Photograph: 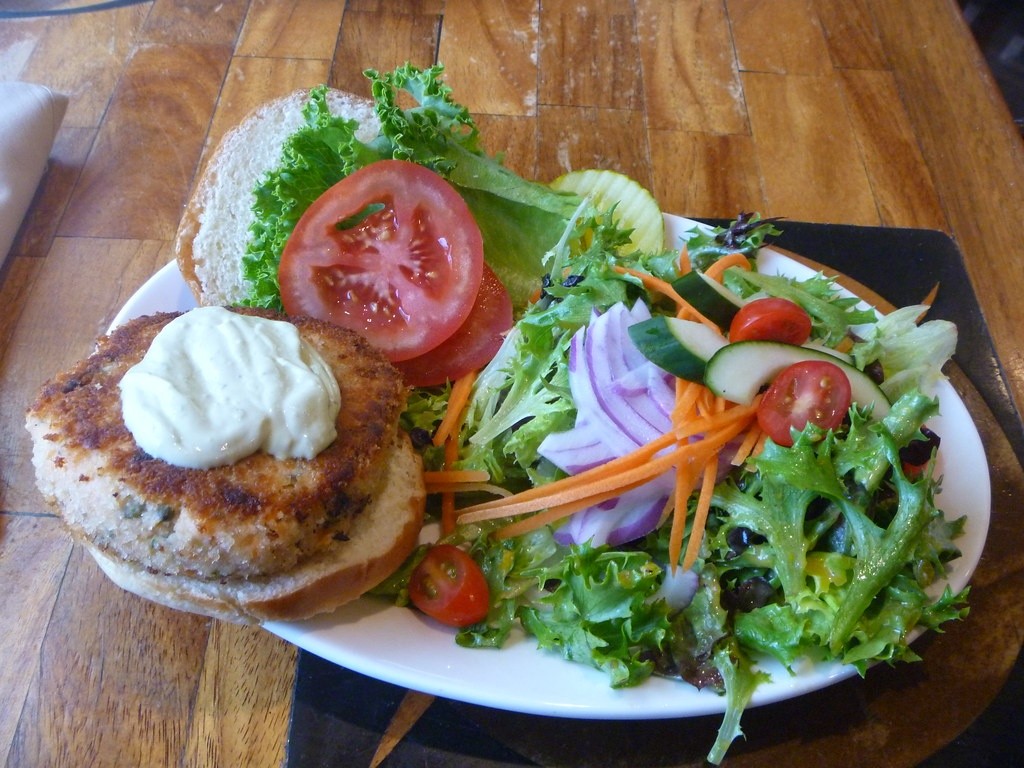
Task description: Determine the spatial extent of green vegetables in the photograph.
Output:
[244,57,973,767]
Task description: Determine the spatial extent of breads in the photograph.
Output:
[175,84,385,308]
[26,301,427,625]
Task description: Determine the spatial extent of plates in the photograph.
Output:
[87,196,996,717]
[274,223,1023,768]
